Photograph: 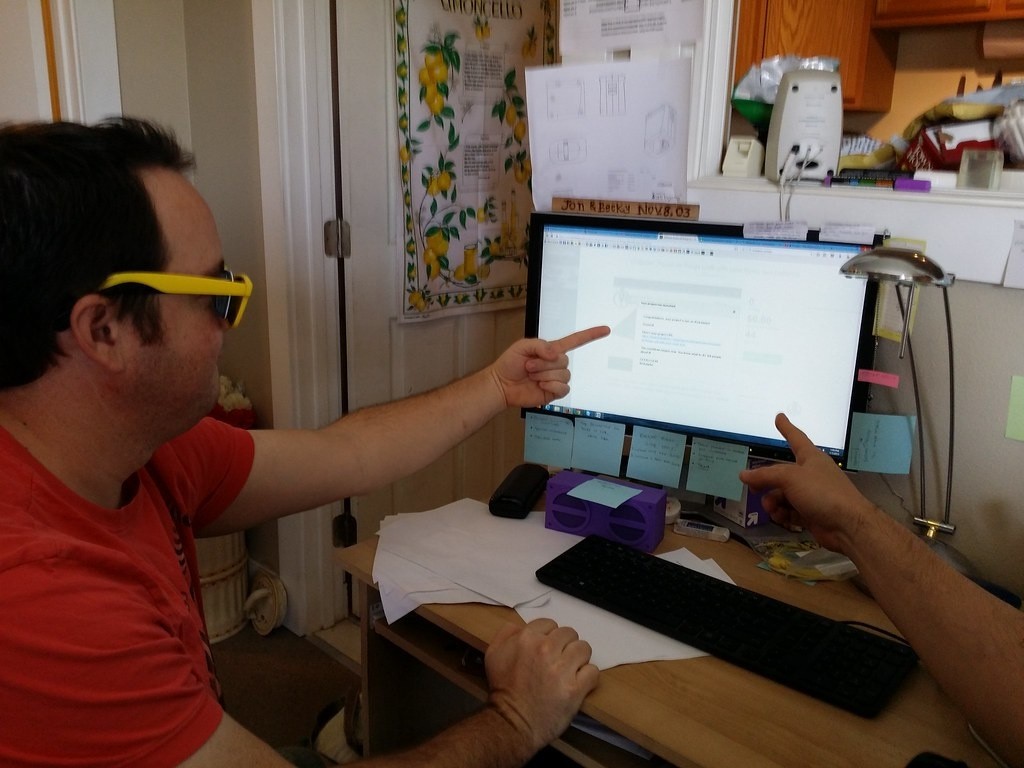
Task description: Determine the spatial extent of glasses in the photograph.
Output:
[99,263,253,329]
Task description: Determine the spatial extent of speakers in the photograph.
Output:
[546,472,666,552]
[765,69,845,181]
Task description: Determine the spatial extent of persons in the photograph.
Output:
[739,412,1023,768]
[1,112,612,768]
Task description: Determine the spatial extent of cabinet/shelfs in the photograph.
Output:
[733,1,1024,112]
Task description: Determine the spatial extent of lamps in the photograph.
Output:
[841,247,959,543]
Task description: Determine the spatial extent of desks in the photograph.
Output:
[333,499,1007,768]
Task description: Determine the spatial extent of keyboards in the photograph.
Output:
[536,534,920,720]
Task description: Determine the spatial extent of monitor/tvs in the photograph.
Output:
[522,212,891,474]
